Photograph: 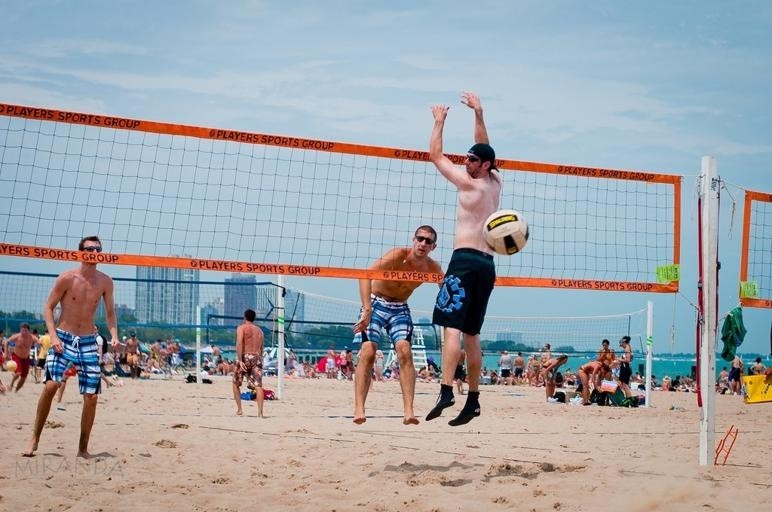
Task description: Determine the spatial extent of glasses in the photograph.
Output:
[467,154,479,162]
[84,246,102,252]
[415,236,435,244]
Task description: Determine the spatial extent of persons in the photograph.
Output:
[0,325,765,407]
[233,309,264,418]
[426,91,503,426]
[21,236,119,459]
[352,225,446,425]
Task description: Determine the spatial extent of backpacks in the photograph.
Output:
[95,335,107,354]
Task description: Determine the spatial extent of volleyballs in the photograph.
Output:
[483,209,529,255]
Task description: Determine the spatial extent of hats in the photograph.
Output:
[468,144,499,172]
